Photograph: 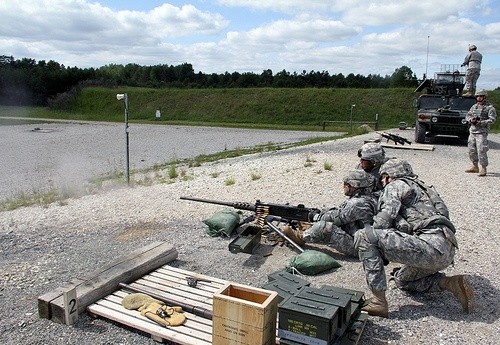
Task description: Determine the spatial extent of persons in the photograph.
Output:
[465,88,496,177]
[285,142,396,260]
[444,45,483,97]
[353,158,475,318]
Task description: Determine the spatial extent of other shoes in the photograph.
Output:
[461,91,473,96]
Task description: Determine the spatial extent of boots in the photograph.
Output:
[360,289,389,318]
[297,221,311,230]
[465,162,479,173]
[284,227,305,245]
[439,275,474,314]
[478,165,486,176]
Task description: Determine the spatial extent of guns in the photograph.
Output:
[179,195,320,226]
[376,130,412,146]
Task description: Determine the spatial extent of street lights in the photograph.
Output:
[425,35,431,79]
[116,93,132,188]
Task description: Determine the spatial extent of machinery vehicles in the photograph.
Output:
[410,63,480,144]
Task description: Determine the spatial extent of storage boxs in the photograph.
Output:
[212,270,366,345]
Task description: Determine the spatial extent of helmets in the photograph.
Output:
[475,88,488,97]
[469,45,476,51]
[380,158,413,179]
[342,171,369,196]
[358,143,384,165]
